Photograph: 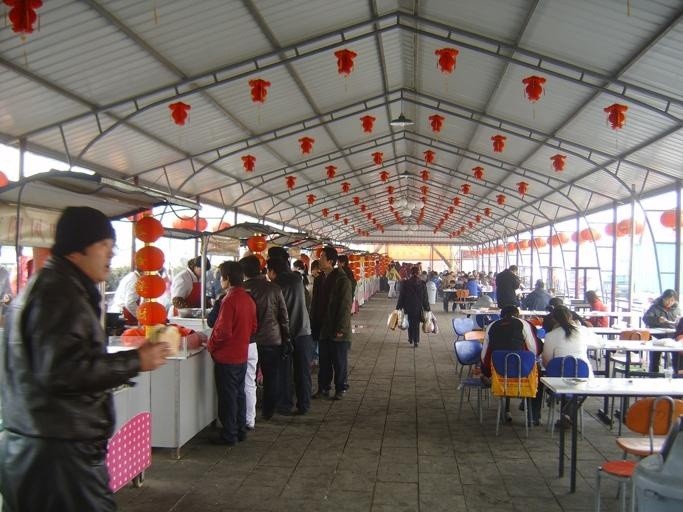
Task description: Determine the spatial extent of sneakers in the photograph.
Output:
[555,418,572,429]
[506,410,512,421]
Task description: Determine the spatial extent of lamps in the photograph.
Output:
[387,89,414,130]
[397,155,414,177]
[391,188,424,235]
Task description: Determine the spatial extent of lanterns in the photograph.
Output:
[3,0,43,33]
[248,79,271,105]
[360,115,375,133]
[0,170,9,189]
[522,76,545,103]
[428,115,444,133]
[335,48,357,79]
[435,48,459,74]
[604,104,627,130]
[169,102,191,126]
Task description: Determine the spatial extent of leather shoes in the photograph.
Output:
[207,429,245,446]
[408,339,418,347]
[334,388,346,400]
[311,389,330,400]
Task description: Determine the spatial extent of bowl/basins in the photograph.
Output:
[175,307,202,318]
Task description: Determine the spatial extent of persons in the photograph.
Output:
[0,207,175,512]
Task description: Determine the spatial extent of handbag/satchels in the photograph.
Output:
[423,310,434,333]
[386,308,409,331]
[420,305,426,323]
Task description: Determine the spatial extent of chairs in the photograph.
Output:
[490,348,538,444]
[540,317,683,510]
[455,342,494,423]
[426,275,640,342]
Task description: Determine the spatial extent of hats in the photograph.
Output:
[50,206,116,258]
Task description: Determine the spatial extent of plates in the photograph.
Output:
[563,378,587,386]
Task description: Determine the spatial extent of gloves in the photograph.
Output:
[279,334,294,360]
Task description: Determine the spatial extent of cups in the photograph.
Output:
[663,369,673,383]
